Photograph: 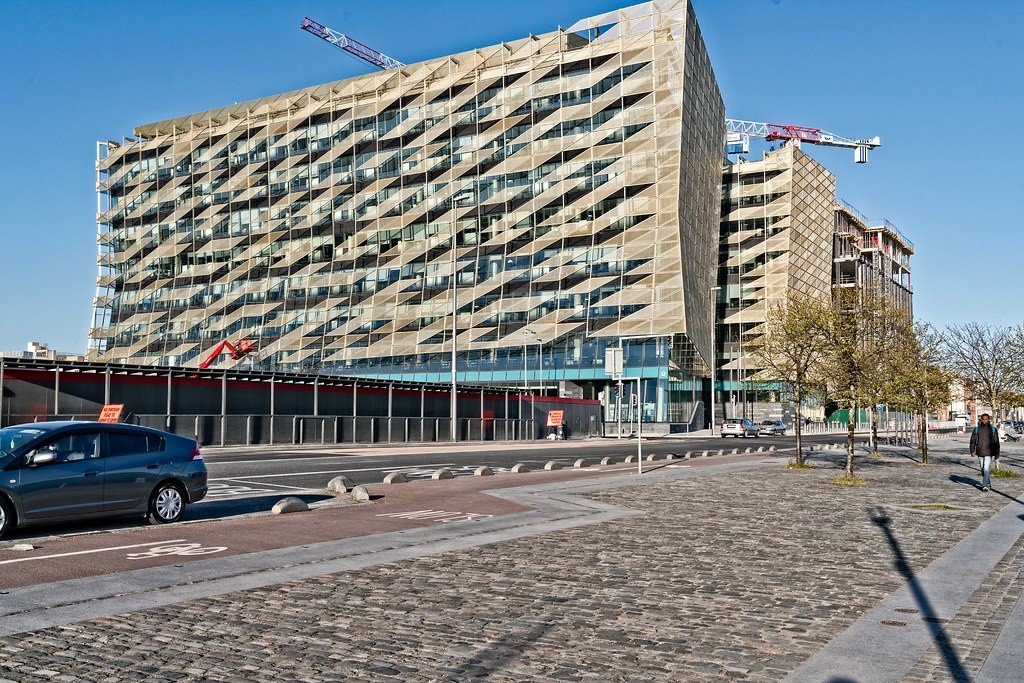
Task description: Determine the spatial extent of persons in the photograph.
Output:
[806,417,813,425]
[1018,419,1024,427]
[970,414,1000,492]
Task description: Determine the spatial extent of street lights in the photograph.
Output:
[710,286,722,435]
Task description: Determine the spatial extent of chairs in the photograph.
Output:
[63,435,91,462]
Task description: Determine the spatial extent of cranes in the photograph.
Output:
[724,118,881,163]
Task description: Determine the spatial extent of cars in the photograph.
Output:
[955,415,970,427]
[759,420,787,436]
[0,421,210,541]
[720,418,760,439]
[1001,420,1024,429]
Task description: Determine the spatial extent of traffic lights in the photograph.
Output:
[633,395,636,405]
[615,384,620,397]
[622,384,626,398]
[668,335,673,349]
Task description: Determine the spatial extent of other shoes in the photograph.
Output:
[983,484,991,492]
